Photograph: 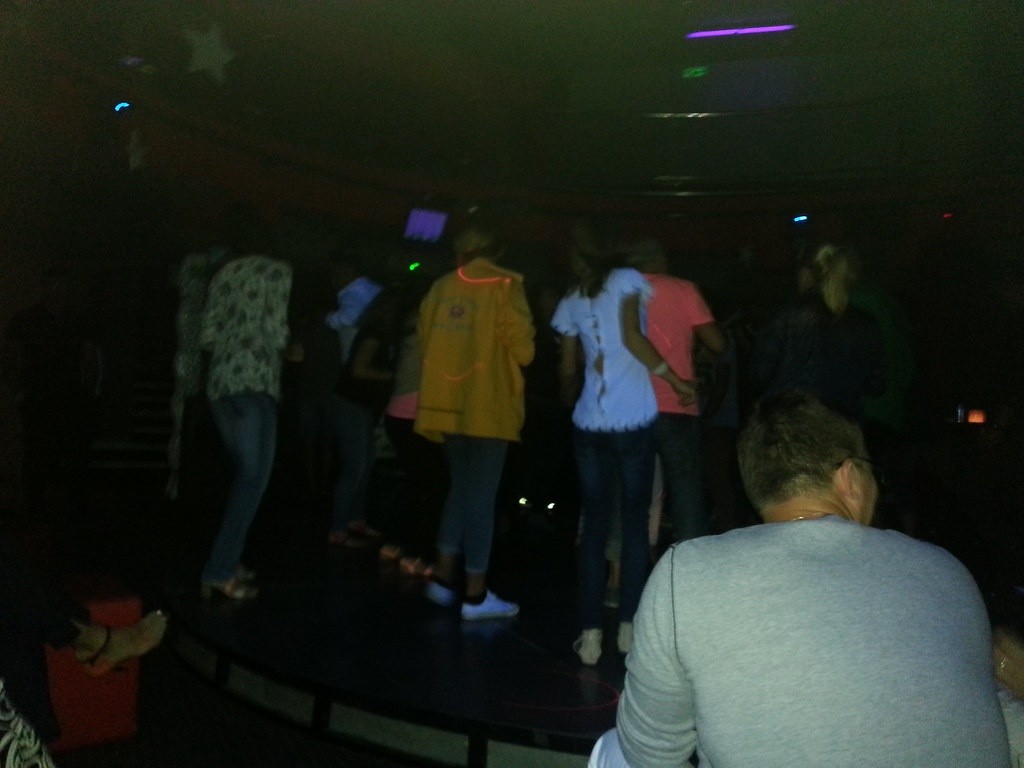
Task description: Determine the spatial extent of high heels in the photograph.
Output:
[201,579,257,600]
[234,566,253,580]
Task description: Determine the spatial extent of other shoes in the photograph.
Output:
[617,621,633,653]
[571,629,603,664]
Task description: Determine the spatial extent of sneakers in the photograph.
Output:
[461,590,520,621]
[421,576,453,607]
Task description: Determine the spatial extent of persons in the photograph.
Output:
[0,215,1024,768]
[584,380,1010,767]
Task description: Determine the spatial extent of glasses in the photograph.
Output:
[840,457,881,482]
